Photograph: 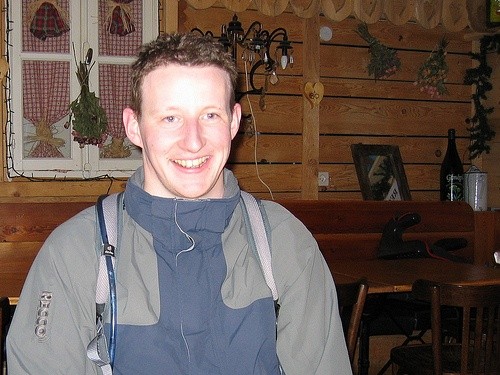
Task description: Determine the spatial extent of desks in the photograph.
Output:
[327,260,500,294]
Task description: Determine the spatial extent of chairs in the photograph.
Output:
[336,284,369,356]
[391,279,500,375]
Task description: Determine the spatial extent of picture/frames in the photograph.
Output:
[350,143,412,201]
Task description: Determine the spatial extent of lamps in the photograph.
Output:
[189,14,294,110]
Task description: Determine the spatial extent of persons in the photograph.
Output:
[5,32,353,375]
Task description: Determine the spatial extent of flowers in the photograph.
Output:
[355,23,401,82]
[413,36,451,98]
[63,43,111,148]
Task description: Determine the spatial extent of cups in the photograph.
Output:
[463,173,488,211]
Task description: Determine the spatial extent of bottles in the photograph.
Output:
[439,128,464,202]
